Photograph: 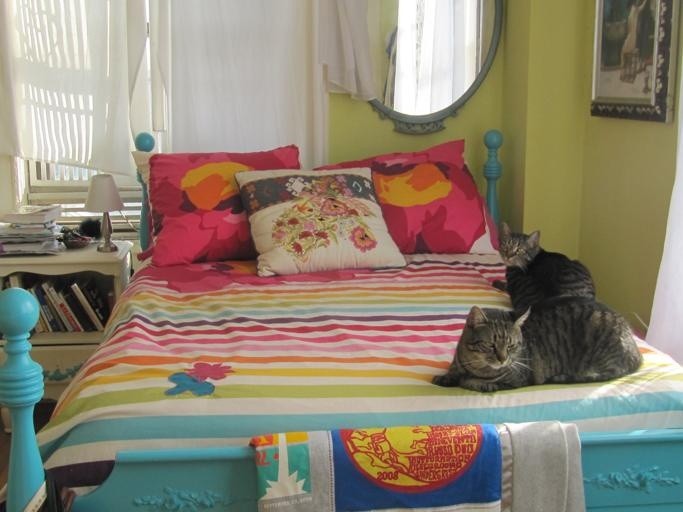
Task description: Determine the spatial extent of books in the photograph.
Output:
[0,273,114,333]
[0,204,59,255]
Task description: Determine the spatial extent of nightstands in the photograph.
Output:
[0,239,135,433]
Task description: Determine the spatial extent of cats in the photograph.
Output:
[491,222,596,311]
[431,295,643,393]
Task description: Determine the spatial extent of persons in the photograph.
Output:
[619,0,648,70]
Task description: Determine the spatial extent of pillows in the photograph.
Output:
[234,167,406,278]
[131,144,301,267]
[313,138,501,256]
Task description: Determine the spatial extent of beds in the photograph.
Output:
[0,128,683,512]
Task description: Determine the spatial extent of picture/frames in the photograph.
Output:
[589,0,680,126]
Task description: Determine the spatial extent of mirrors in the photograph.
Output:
[358,0,504,135]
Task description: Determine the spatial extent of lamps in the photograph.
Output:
[83,173,126,253]
